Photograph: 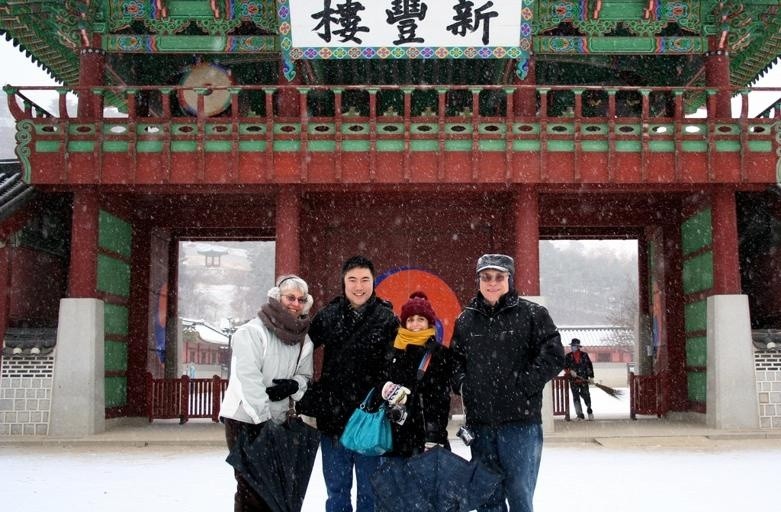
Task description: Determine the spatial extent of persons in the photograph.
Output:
[446,252,567,512]
[564,338,596,421]
[359,291,451,461]
[218,275,314,512]
[307,254,407,511]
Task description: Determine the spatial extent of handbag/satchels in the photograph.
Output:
[339,387,394,458]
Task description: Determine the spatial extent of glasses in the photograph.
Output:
[478,273,509,282]
[280,293,308,304]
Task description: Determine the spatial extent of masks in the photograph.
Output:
[572,345,577,352]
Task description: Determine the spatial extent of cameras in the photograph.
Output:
[456,426,476,447]
[390,404,409,426]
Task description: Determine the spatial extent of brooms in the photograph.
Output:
[575,374,625,401]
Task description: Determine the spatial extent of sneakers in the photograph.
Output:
[575,414,594,422]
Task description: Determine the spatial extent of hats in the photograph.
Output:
[401,291,436,329]
[569,338,583,348]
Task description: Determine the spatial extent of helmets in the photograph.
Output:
[475,253,515,274]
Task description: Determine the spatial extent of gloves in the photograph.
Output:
[590,378,594,384]
[571,370,576,377]
[265,379,299,402]
[382,381,412,426]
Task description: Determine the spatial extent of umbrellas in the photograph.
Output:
[222,392,325,512]
[366,393,507,512]
